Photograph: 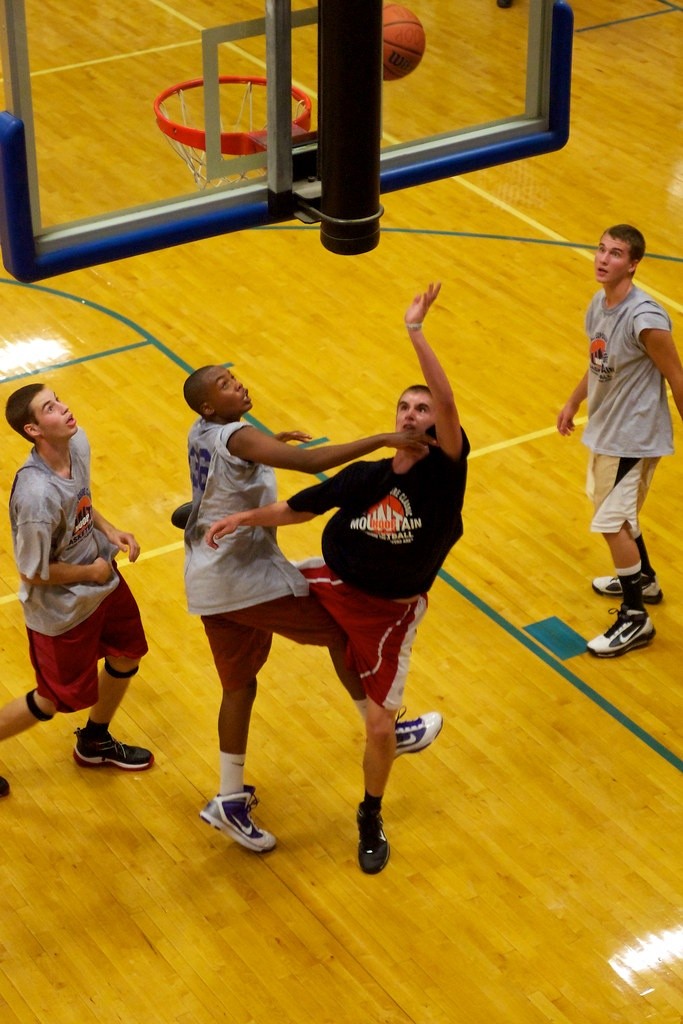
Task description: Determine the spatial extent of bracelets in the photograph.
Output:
[409,323,421,329]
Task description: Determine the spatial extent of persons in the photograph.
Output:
[0,384,155,798]
[556,224,683,657]
[182,366,443,854]
[170,283,472,875]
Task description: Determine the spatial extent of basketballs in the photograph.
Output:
[382,3,426,81]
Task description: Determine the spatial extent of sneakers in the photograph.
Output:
[592,571,663,605]
[357,802,390,875]
[393,706,443,760]
[171,501,192,529]
[0,776,10,797]
[199,784,276,853]
[586,604,656,657]
[73,726,154,771]
